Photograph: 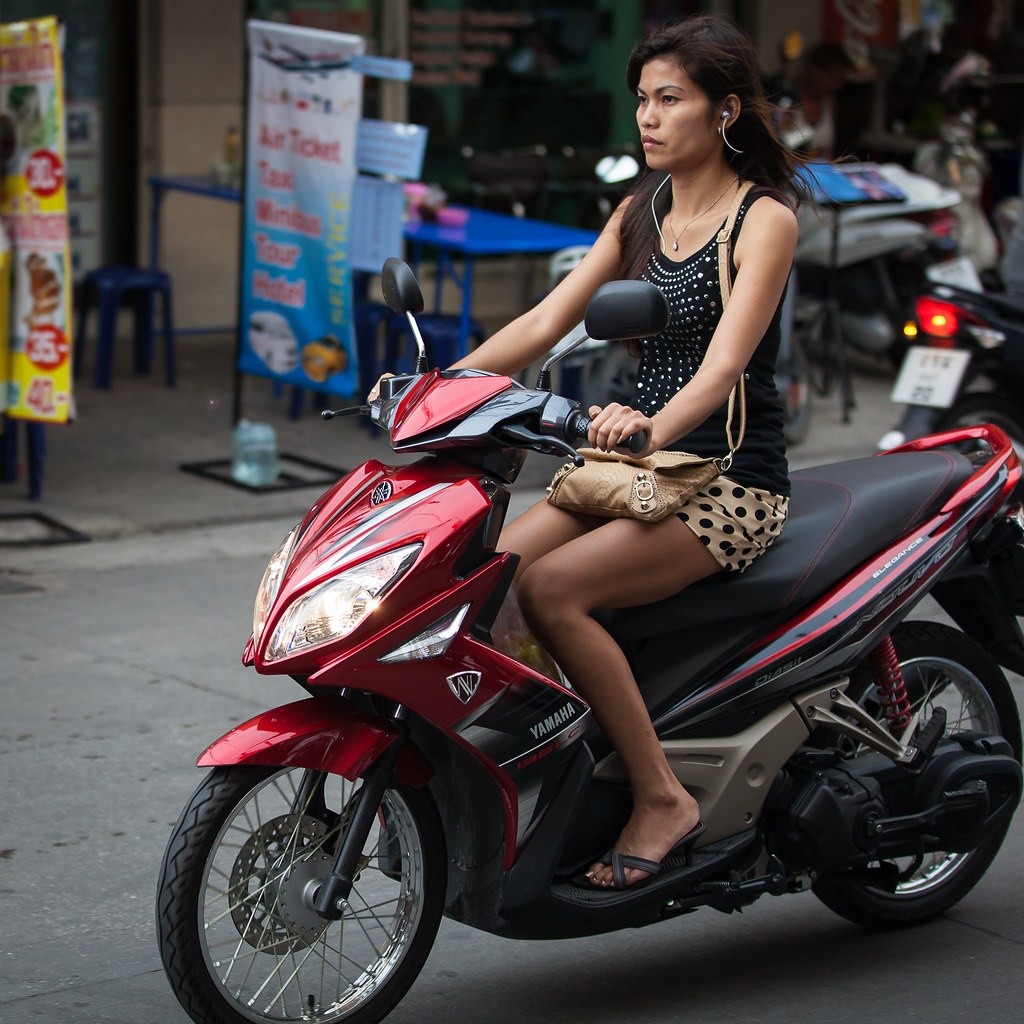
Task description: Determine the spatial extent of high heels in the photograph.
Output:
[574,807,708,890]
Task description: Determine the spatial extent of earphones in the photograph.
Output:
[721,110,730,119]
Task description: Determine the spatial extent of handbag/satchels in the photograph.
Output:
[548,440,724,524]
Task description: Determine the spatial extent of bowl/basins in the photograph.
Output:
[438,209,466,225]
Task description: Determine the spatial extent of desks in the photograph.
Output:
[142,175,599,420]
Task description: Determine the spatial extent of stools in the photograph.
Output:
[0,262,486,501]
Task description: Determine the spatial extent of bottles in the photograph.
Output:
[230,421,280,487]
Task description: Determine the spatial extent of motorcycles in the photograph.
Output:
[153,257,1024,1024]
[878,264,1024,519]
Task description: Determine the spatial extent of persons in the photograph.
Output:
[770,0,1024,353]
[461,36,614,148]
[367,17,864,889]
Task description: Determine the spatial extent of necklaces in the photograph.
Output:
[669,177,738,251]
